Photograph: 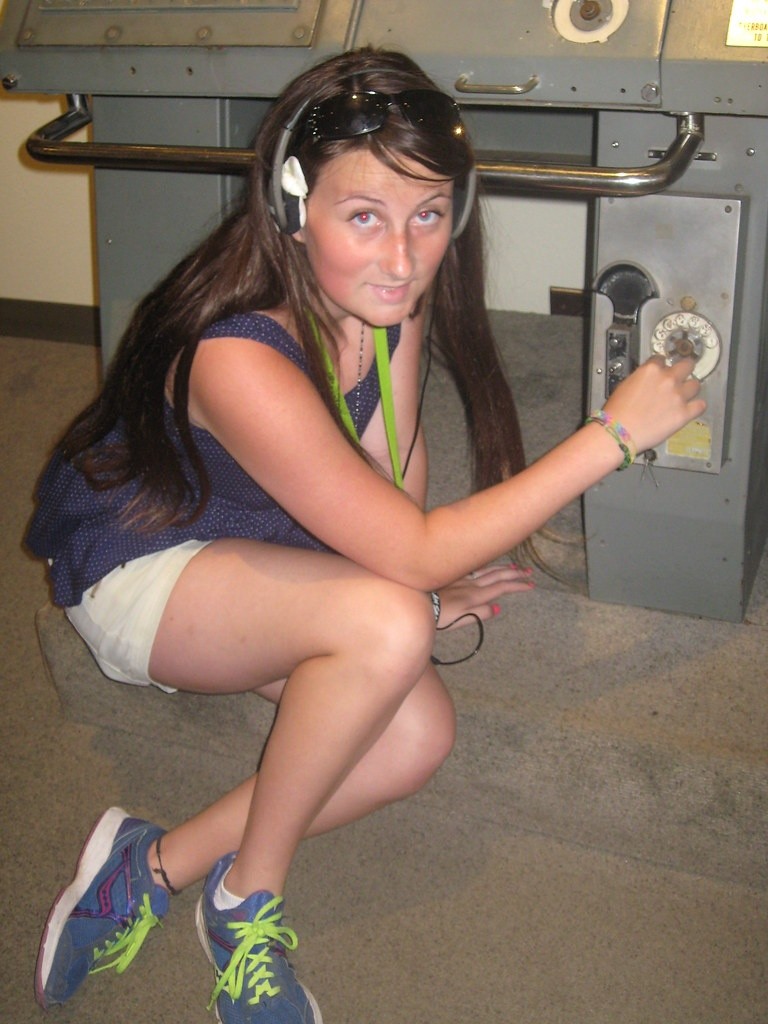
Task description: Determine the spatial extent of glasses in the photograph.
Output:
[283,89,462,159]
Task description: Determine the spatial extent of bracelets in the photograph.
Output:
[585,410,637,472]
[431,590,442,618]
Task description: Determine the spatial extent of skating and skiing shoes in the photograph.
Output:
[35,806,171,1013]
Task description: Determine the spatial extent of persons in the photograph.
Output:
[22,48,707,1024]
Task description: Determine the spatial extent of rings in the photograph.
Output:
[471,572,477,579]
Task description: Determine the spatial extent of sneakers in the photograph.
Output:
[195,850,323,1024]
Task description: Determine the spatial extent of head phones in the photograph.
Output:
[264,66,476,239]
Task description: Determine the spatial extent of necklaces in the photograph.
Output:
[351,316,365,435]
